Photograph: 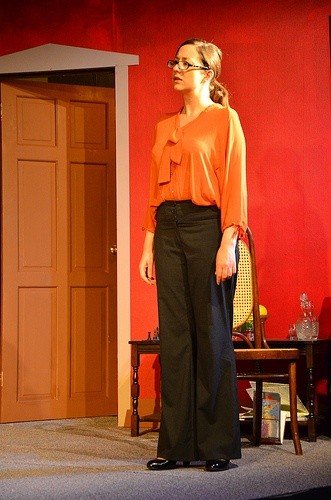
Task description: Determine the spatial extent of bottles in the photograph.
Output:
[296,291,313,342]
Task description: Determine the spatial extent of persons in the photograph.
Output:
[139,38,248,471]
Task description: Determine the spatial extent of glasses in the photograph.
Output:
[167,60,209,70]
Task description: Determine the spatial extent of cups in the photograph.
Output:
[312,321,319,341]
[288,323,299,341]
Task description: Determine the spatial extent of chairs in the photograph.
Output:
[233,227,304,456]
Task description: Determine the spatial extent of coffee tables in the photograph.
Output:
[127,340,331,447]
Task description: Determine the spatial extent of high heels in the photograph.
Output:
[147,458,191,470]
[205,459,229,471]
